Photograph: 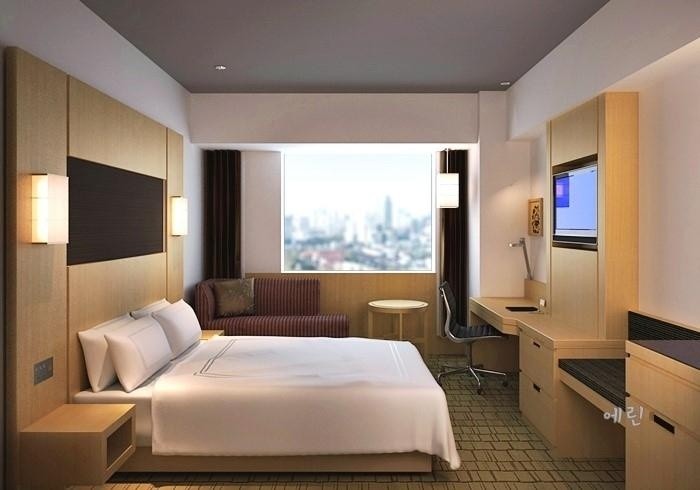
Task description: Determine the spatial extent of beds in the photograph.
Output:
[72,336,432,473]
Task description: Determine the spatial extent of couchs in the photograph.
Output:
[194,278,348,338]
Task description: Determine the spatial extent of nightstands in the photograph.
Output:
[200,328,224,340]
[18,404,137,489]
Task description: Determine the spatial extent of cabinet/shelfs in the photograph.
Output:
[517,316,627,460]
[624,337,700,489]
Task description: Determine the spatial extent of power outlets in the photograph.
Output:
[33,356,54,385]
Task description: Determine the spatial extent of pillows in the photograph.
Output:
[77,312,138,393]
[104,313,175,393]
[213,278,254,317]
[151,298,203,361]
[130,298,171,320]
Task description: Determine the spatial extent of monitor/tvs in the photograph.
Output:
[550,154,598,251]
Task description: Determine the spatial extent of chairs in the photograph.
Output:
[436,281,510,395]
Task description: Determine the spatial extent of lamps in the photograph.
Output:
[215,65,226,70]
[500,81,510,86]
[171,196,188,236]
[437,147,460,208]
[31,172,69,244]
[509,237,531,279]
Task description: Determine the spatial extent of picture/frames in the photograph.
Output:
[528,198,543,237]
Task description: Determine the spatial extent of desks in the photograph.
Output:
[368,299,428,357]
[471,296,542,335]
